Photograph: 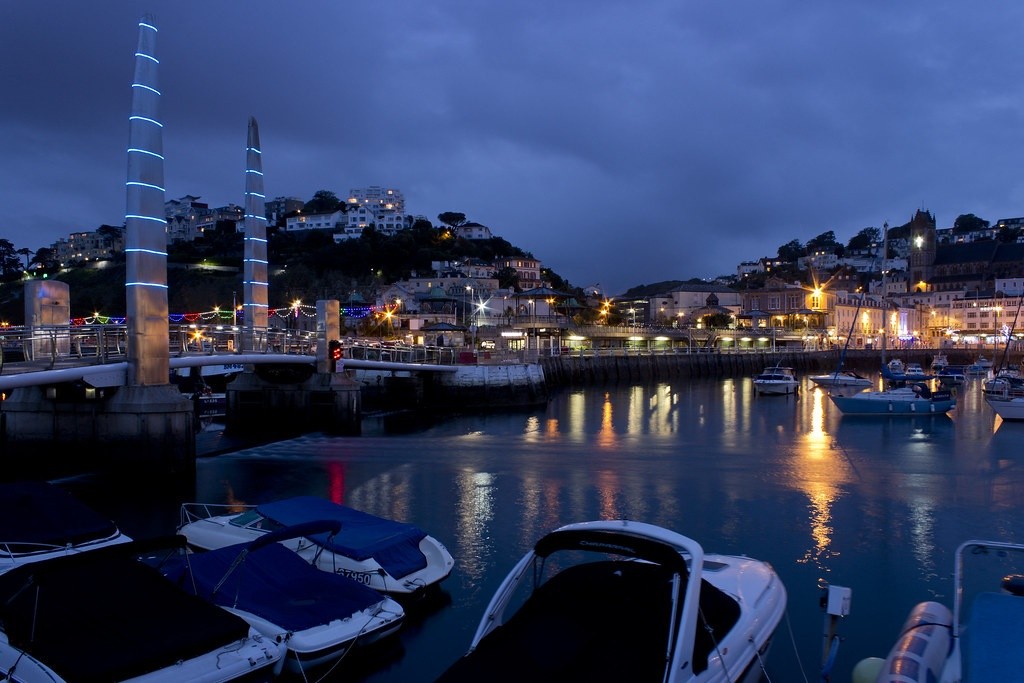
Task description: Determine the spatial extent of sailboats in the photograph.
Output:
[826,222,955,416]
[983,284,1024,424]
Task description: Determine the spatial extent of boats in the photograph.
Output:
[848,539,1023,683]
[177,498,455,603]
[0,468,291,683]
[426,514,787,683]
[752,365,799,395]
[139,537,407,675]
[810,369,872,387]
[880,354,994,387]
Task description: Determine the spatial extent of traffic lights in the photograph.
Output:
[330,340,342,361]
